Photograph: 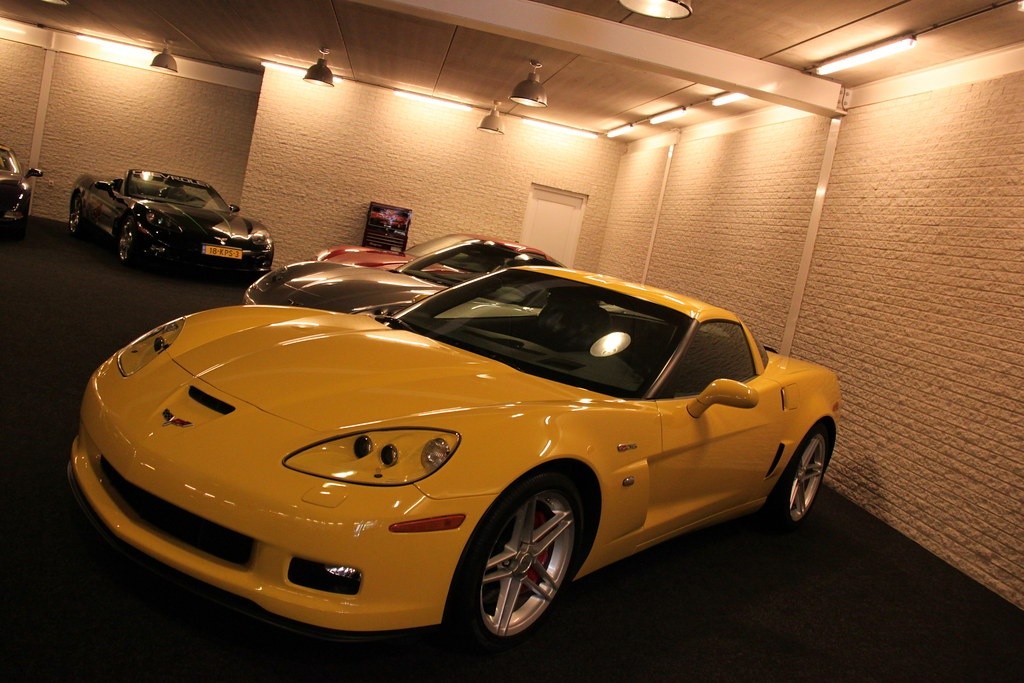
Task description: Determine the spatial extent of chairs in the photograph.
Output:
[109,179,143,196]
[159,187,192,202]
[462,248,496,269]
[539,296,612,358]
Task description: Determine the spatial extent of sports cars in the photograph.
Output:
[69,169,275,272]
[244,232,626,320]
[68,264,840,654]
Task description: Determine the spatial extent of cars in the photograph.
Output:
[0,145,43,239]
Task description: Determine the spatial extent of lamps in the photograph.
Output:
[508,59,548,108]
[478,98,504,136]
[565,306,633,357]
[616,0,693,20]
[149,40,178,73]
[648,105,684,124]
[302,47,335,88]
[712,91,748,106]
[815,32,918,75]
[606,122,631,138]
